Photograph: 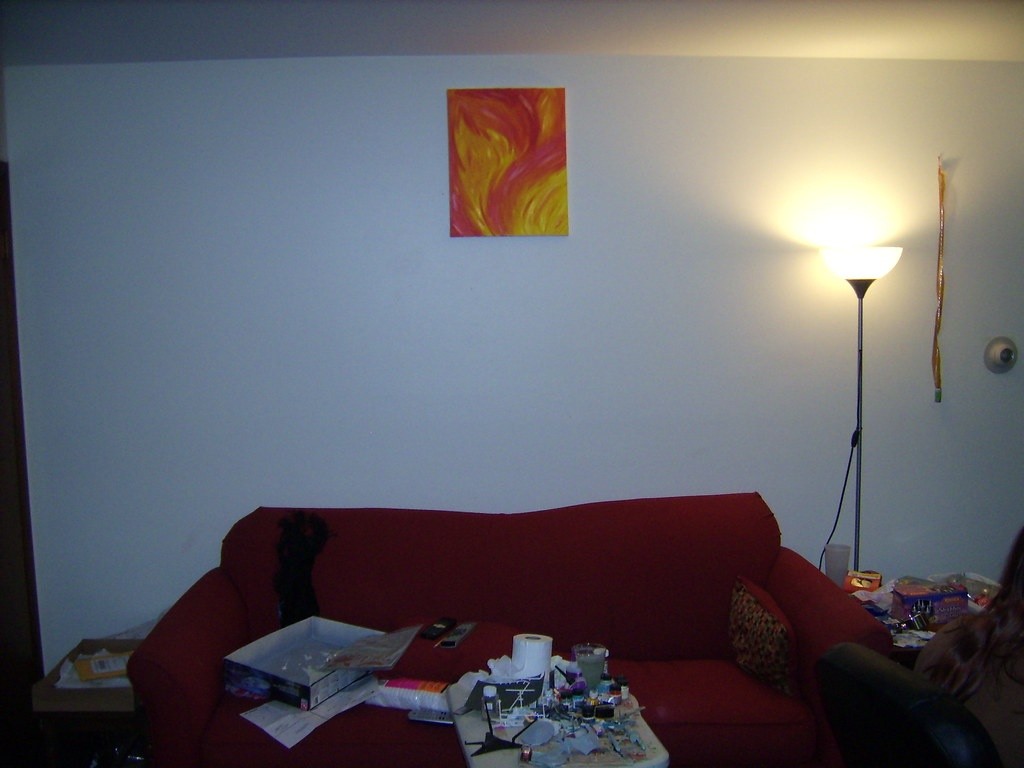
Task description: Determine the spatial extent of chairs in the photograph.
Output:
[814,641,1005,768]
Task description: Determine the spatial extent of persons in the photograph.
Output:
[914,525,1024,768]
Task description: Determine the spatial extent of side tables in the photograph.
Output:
[32,638,145,768]
[888,608,977,671]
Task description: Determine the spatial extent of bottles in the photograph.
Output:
[541,673,628,724]
[483,687,499,721]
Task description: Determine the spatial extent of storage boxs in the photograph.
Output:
[223,615,388,710]
[892,584,968,625]
[843,571,881,602]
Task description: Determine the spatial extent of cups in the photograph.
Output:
[825,544,851,589]
[574,644,606,690]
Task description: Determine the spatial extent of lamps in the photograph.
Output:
[820,246,903,572]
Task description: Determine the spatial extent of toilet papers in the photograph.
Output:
[512,634,553,673]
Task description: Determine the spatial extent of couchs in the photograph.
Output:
[126,491,894,768]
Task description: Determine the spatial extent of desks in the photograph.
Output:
[446,684,670,768]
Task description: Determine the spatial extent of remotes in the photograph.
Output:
[440,621,479,648]
[408,709,454,725]
[420,616,458,641]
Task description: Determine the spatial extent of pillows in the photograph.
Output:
[374,616,524,683]
[730,574,800,700]
